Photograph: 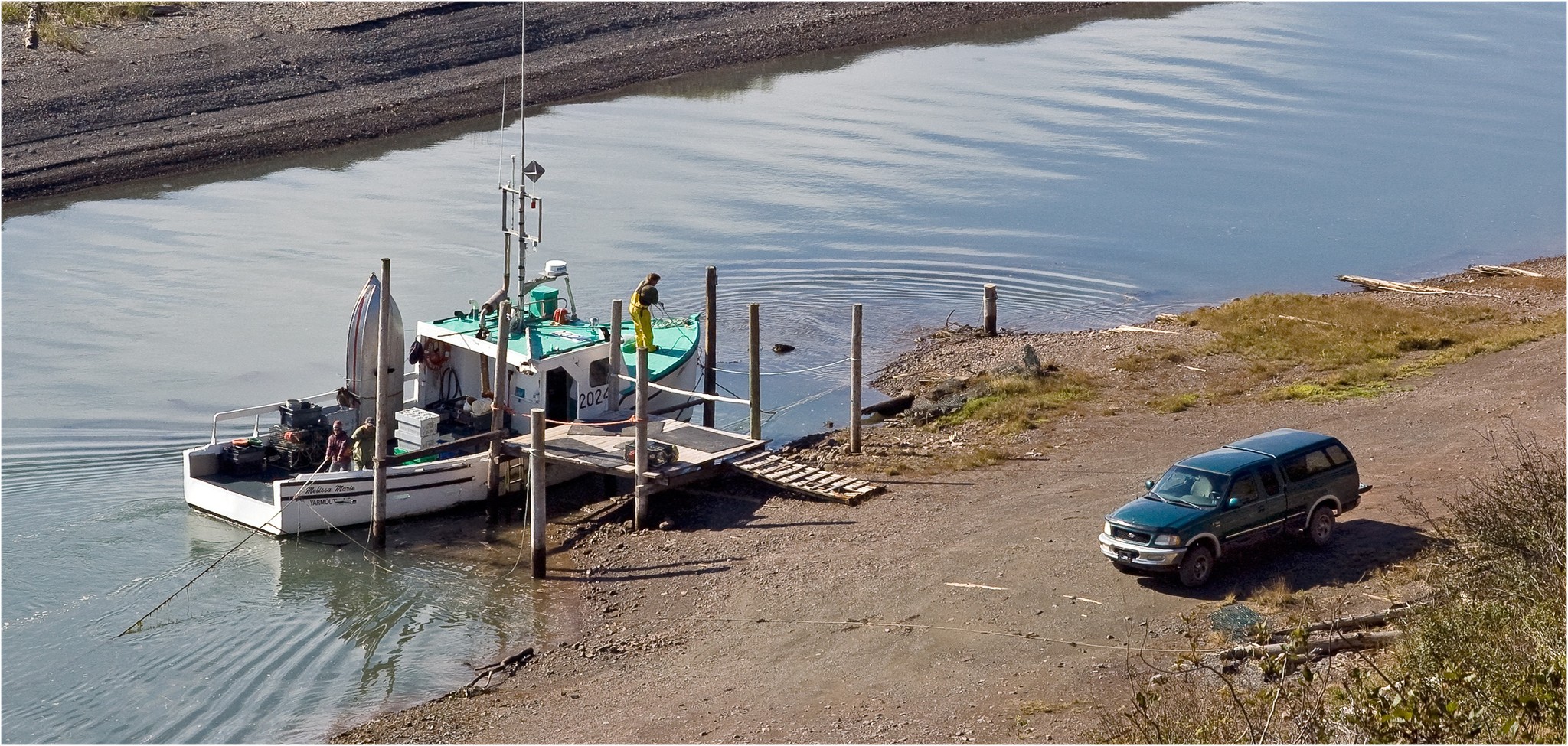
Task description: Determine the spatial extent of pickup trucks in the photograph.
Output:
[1099,427,1372,589]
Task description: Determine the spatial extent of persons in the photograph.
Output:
[628,272,661,352]
[324,420,353,472]
[350,417,376,471]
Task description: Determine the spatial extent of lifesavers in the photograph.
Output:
[423,337,451,371]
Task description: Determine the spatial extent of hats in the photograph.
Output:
[366,416,374,426]
[333,421,342,428]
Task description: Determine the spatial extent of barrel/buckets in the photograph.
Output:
[463,396,477,411]
[248,438,263,446]
[286,399,302,410]
[232,439,248,447]
[472,398,492,414]
[437,440,459,460]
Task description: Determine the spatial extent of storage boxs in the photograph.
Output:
[445,395,511,435]
[394,405,441,467]
[279,401,323,429]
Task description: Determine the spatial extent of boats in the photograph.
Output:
[182,1,704,537]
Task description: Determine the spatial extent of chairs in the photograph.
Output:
[1190,473,1215,498]
